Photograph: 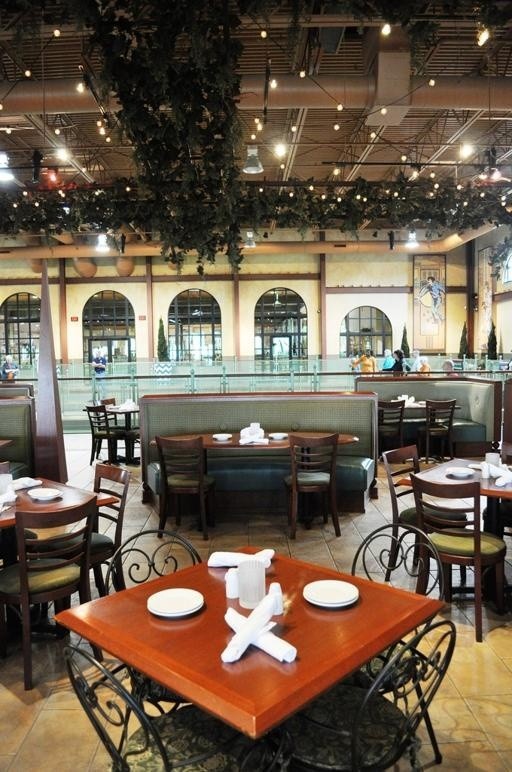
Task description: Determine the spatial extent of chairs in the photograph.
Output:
[382,444,466,594]
[60,646,295,771]
[26,462,131,663]
[1,495,104,691]
[1,382,512,539]
[408,471,508,643]
[103,530,205,714]
[351,524,445,695]
[267,620,458,771]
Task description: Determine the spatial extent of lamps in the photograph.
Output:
[0,0,511,278]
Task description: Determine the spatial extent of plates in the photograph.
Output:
[418,402,425,405]
[211,433,232,440]
[145,588,205,618]
[268,432,288,440]
[445,466,475,478]
[301,580,359,609]
[28,489,64,502]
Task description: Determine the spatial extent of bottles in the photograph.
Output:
[225,568,239,599]
[270,582,284,615]
[479,458,501,479]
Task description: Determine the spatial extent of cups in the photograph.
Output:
[238,560,266,609]
[402,394,407,400]
[485,452,498,467]
[240,421,265,440]
[0,472,15,503]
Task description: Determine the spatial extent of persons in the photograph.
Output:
[420,277,445,323]
[483,283,491,336]
[0,355,18,385]
[94,350,106,400]
[215,353,221,367]
[348,346,459,378]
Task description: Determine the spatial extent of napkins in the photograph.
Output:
[208,549,275,598]
[220,581,297,663]
